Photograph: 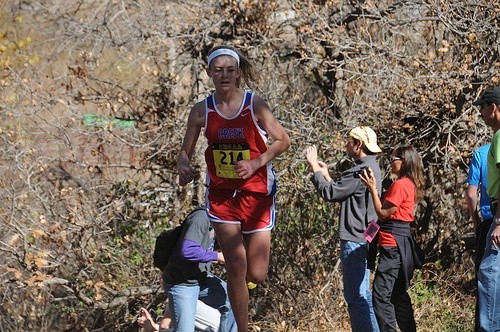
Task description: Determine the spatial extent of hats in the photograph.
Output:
[472,86,500,106]
[349,125,383,153]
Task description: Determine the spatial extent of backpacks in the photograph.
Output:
[152,206,213,272]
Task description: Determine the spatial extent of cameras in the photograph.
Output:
[353,166,370,180]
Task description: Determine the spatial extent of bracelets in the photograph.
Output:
[368,187,379,193]
[493,216,500,225]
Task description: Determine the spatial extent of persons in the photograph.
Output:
[306,127,382,332]
[472,85,500,332]
[175,46,291,332]
[164,205,244,331]
[468,143,491,330]
[358,146,423,332]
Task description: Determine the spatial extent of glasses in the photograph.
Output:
[389,156,403,163]
